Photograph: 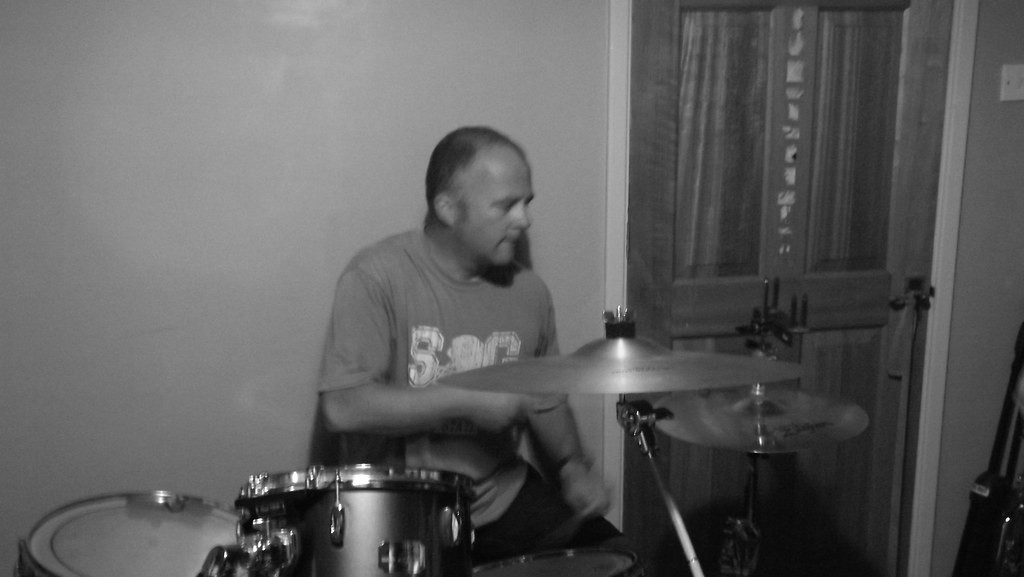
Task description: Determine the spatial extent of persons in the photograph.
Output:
[317,127,624,577]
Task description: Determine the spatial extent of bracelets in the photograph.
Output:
[556,456,592,476]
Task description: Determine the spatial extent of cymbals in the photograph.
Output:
[436,331,819,398]
[653,390,871,456]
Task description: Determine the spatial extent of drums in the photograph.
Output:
[237,463,480,577]
[28,489,242,577]
[473,546,647,577]
